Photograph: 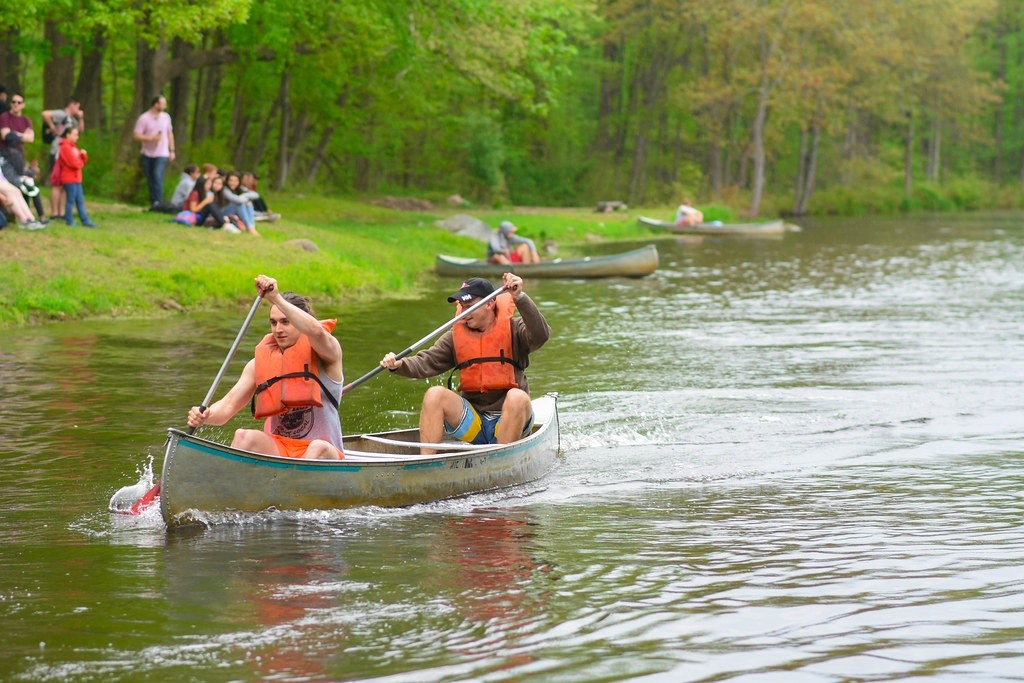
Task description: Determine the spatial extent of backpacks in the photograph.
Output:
[42,108,69,144]
[50,150,62,187]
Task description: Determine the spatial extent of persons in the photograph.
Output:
[132,95,176,206]
[486,221,540,265]
[170,163,280,238]
[42,96,85,220]
[676,199,704,227]
[187,274,344,461]
[58,126,96,227]
[380,273,550,455]
[0,85,50,231]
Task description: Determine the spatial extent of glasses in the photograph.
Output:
[11,100,23,104]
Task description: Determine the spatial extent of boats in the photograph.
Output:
[161,392,560,527]
[436,244,658,280]
[637,216,784,238]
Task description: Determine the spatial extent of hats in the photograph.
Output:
[447,278,496,303]
[6,132,22,146]
[510,226,518,232]
[20,177,40,197]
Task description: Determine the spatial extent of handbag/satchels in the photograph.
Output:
[175,210,202,227]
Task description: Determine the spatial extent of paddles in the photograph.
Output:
[131,278,276,514]
[342,282,518,394]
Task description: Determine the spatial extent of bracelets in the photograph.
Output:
[79,116,84,121]
[170,149,175,152]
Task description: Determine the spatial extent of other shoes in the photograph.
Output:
[18,220,46,230]
[39,218,48,224]
[268,213,281,222]
[248,227,257,235]
[222,223,241,234]
[237,219,246,230]
